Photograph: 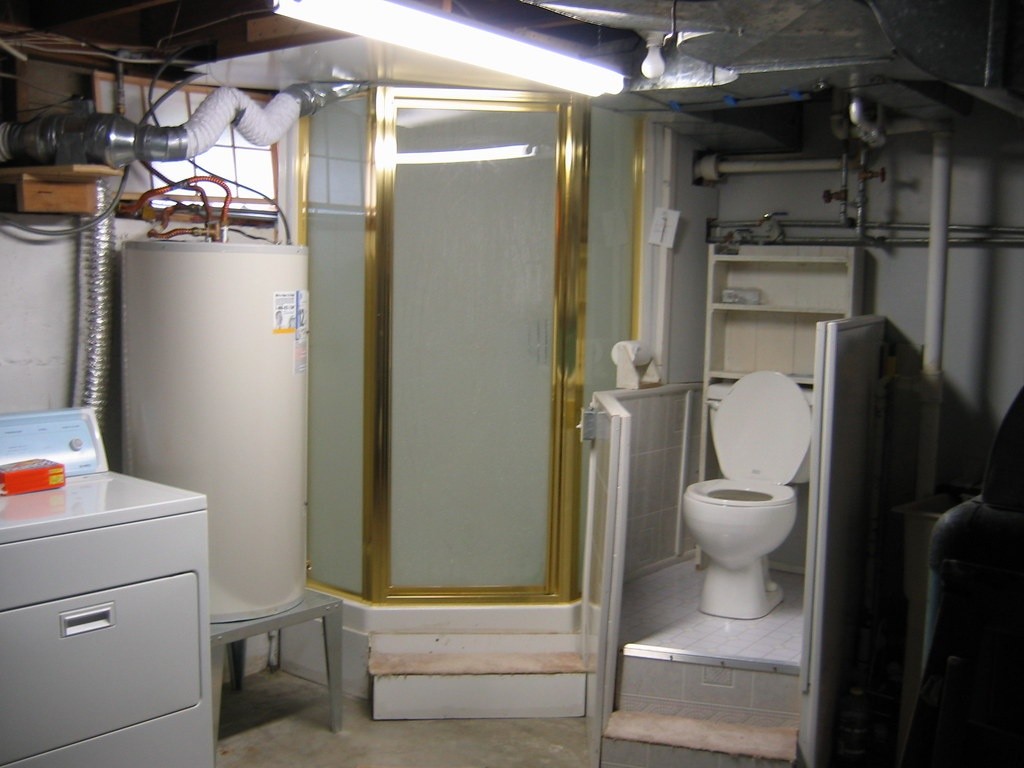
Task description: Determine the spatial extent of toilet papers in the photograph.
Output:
[610,340,651,390]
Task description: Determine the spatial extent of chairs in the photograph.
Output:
[908,393,1024,768]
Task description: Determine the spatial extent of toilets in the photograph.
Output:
[682,369,814,620]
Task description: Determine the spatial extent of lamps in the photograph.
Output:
[275,1,631,96]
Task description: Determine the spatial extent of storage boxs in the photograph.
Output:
[0,459,65,495]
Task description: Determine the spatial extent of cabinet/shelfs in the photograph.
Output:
[685,247,867,574]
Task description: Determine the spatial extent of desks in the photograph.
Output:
[211,590,345,751]
